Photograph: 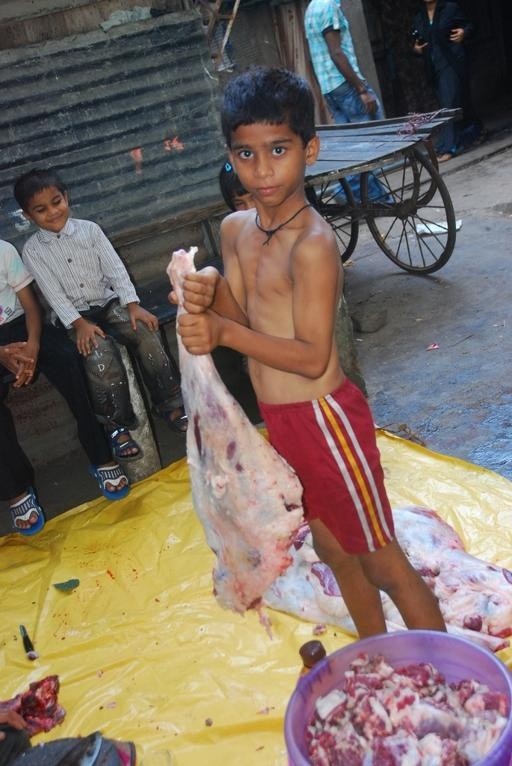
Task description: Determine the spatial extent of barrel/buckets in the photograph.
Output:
[284,629,512,766]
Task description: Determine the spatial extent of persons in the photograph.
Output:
[219,159,256,211]
[0,239,130,536]
[304,0,394,204]
[413,0,488,162]
[168,67,447,640]
[14,171,187,460]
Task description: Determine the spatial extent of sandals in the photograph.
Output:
[109,428,143,462]
[151,404,188,434]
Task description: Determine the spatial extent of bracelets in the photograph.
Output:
[358,86,368,95]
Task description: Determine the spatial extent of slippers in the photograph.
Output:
[89,464,129,500]
[10,486,45,536]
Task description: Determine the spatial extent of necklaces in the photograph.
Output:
[255,203,313,249]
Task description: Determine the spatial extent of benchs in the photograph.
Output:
[4,199,237,383]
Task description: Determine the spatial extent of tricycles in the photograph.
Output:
[302,105,463,275]
